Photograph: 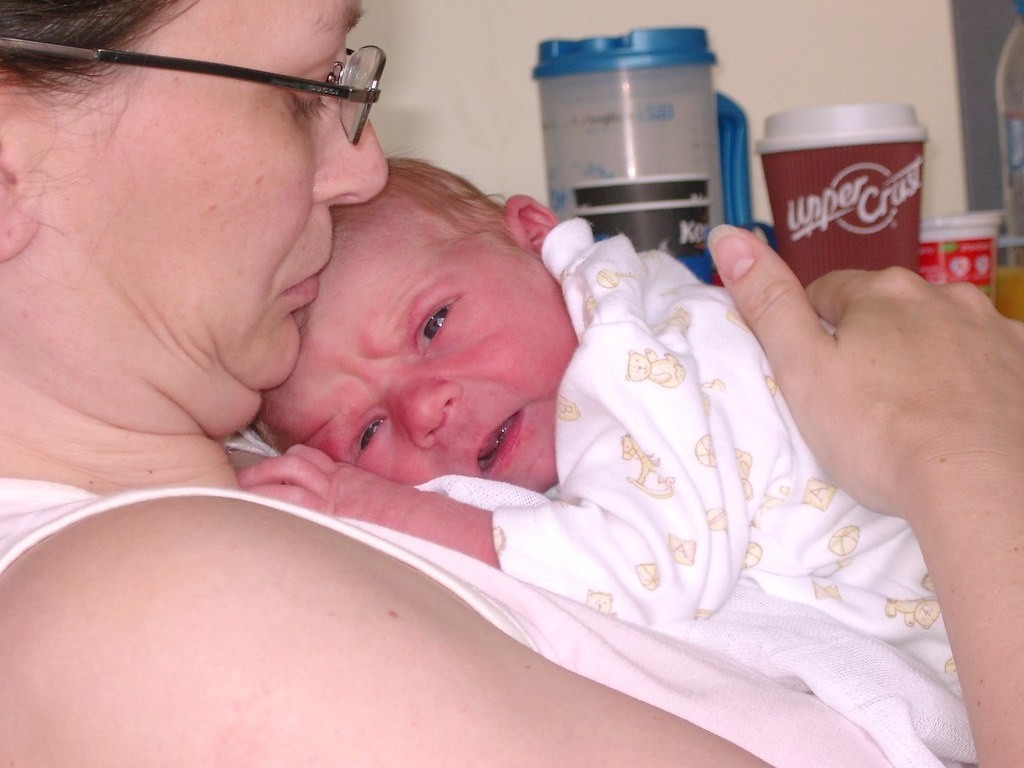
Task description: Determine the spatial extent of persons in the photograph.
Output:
[0,0,1024,768]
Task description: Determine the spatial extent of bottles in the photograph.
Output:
[996,0,1024,267]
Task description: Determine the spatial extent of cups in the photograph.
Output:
[918,216,998,312]
[531,27,724,287]
[755,103,930,291]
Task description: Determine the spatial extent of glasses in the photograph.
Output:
[0,37,386,144]
